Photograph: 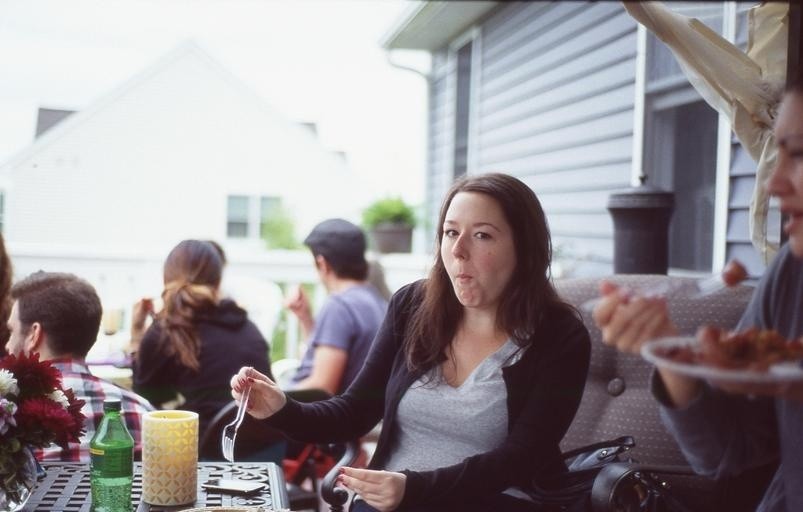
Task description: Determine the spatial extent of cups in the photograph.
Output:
[140,409,198,505]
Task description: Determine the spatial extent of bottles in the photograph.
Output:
[89,399,132,512]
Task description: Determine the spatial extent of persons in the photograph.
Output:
[229,172,591,512]
[592,69,803,512]
[721,259,746,286]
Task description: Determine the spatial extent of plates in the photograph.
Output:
[638,335,802,388]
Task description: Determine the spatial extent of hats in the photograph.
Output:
[303,219,370,272]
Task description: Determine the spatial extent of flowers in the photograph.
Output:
[0,348,92,509]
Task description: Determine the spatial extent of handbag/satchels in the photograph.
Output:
[527,436,634,512]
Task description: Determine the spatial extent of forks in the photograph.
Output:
[580,274,728,313]
[222,367,255,461]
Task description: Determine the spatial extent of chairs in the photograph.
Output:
[196,389,332,512]
[316,269,769,512]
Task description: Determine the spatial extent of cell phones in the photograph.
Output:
[201,477,265,496]
